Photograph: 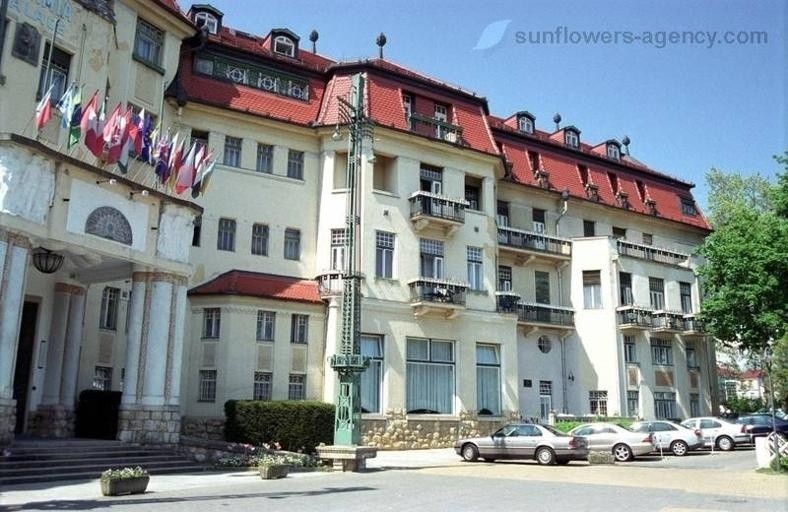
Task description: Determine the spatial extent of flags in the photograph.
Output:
[34,81,218,199]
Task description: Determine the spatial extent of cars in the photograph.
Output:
[455,423,657,465]
[629,414,788,456]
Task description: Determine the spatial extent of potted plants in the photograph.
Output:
[100,466,150,495]
[258,454,291,479]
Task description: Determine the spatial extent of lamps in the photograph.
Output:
[32,246,65,274]
